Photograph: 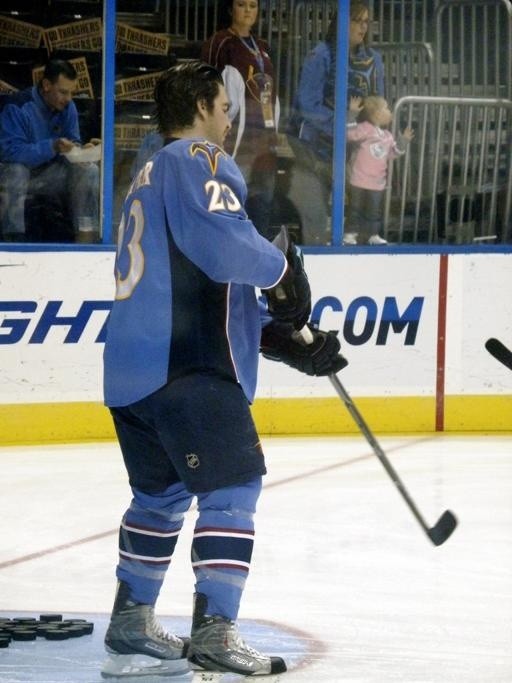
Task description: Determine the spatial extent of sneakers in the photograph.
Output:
[343,232,359,245]
[365,233,387,245]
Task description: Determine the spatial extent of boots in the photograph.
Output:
[104,580,191,660]
[187,589,288,677]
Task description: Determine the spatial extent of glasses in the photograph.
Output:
[351,15,371,25]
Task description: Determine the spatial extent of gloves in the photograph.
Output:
[259,319,348,376]
[260,224,311,331]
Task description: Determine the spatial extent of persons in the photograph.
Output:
[200,0,281,241]
[343,93,416,244]
[284,0,386,245]
[0,56,100,243]
[101,60,350,676]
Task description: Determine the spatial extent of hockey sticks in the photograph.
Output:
[256,285,458,546]
[485,337,511,369]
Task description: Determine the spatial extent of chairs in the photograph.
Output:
[0,0,178,243]
